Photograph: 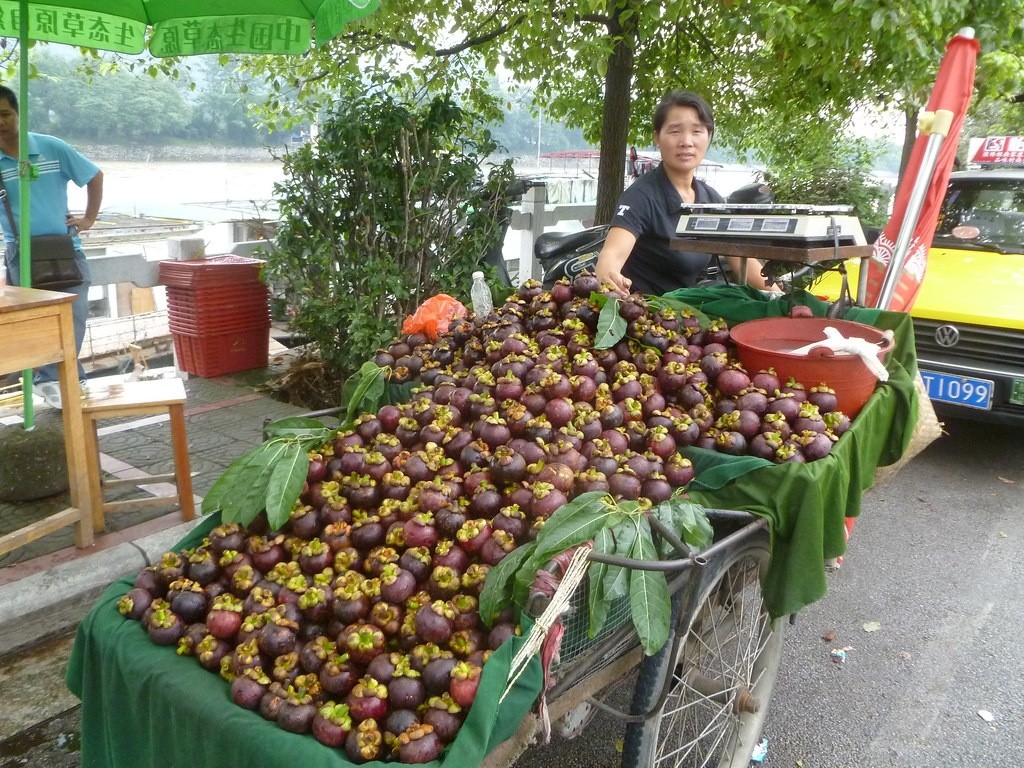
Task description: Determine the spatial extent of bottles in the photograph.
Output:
[0,232,7,297]
[470,271,494,323]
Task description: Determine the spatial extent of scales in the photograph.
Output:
[674,202,868,247]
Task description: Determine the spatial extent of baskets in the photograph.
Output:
[159,254,273,378]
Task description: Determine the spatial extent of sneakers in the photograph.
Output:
[79,380,87,399]
[34,381,62,409]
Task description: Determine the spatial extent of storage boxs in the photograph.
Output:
[158,253,272,378]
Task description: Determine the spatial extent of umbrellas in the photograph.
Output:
[0,0,381,432]
[862,24,978,313]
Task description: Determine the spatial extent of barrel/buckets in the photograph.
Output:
[728,306,895,422]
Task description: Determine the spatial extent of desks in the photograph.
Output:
[0,285,92,557]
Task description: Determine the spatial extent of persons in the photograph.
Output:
[592,92,786,305]
[0,86,103,412]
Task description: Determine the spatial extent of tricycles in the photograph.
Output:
[62,250,940,768]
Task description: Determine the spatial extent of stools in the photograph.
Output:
[61,378,195,532]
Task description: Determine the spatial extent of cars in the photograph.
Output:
[799,134,1024,427]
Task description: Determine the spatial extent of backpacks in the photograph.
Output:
[7,235,83,289]
